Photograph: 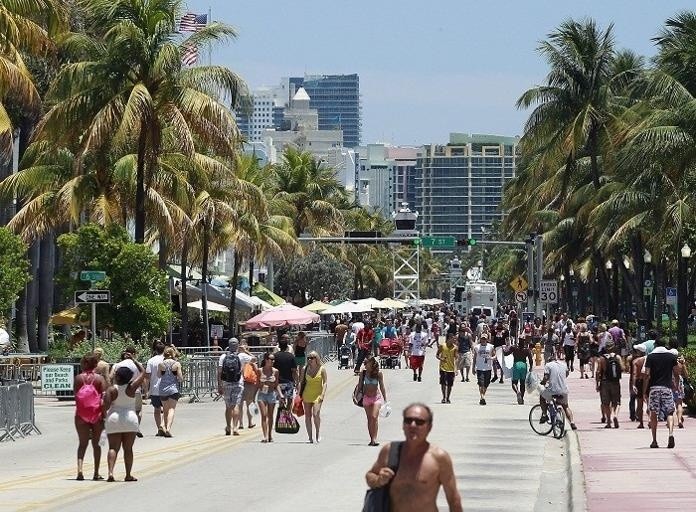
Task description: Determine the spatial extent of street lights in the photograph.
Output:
[621,257,635,316]
[643,249,654,322]
[680,244,692,348]
[561,260,614,318]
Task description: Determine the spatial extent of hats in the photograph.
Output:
[229,338,239,352]
[481,334,488,338]
[633,343,646,353]
[605,340,614,350]
[249,402,259,416]
[379,402,391,417]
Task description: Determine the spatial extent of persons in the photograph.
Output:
[207,314,327,444]
[358,358,386,446]
[72,342,183,481]
[646,337,679,448]
[329,302,687,429]
[366,403,463,511]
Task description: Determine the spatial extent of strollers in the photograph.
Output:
[337,342,354,371]
[379,337,404,370]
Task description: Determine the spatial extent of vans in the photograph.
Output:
[470,305,494,323]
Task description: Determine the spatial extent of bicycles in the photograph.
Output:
[528,384,566,439]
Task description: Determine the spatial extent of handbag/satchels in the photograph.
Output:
[353,384,363,406]
[243,361,257,384]
[276,406,299,433]
[300,382,305,395]
[362,485,390,512]
[525,371,537,393]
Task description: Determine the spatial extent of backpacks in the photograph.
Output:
[221,352,241,382]
[600,353,620,381]
[76,373,103,424]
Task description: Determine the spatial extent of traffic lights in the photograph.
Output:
[458,238,476,247]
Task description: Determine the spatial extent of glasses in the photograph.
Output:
[404,417,425,425]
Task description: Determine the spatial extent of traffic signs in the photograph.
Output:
[74,290,111,305]
[540,279,558,304]
[79,270,106,283]
[422,236,456,248]
